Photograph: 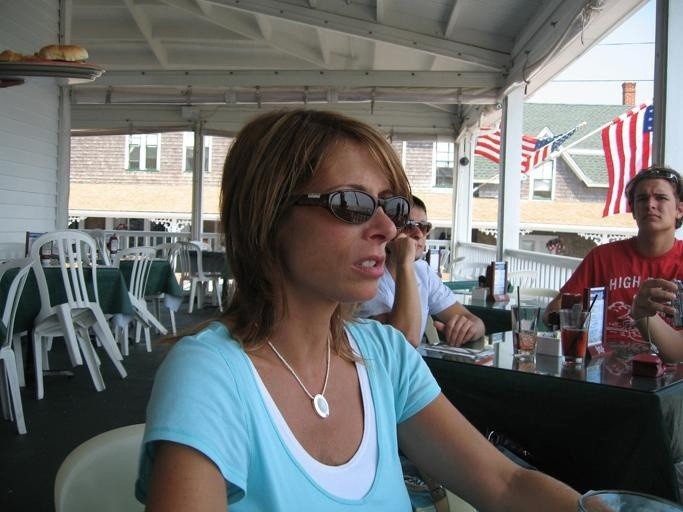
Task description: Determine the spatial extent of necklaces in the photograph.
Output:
[252,321,331,418]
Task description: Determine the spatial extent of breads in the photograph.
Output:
[0,45,88,61]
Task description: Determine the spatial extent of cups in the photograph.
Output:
[577,489,683,512]
[558,309,591,365]
[510,305,540,363]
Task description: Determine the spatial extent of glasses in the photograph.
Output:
[402,219,432,236]
[294,191,410,230]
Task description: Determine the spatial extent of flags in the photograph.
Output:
[602,101,655,218]
[473,124,582,174]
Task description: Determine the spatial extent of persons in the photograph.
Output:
[634,276,683,363]
[353,194,483,348]
[135,108,614,512]
[544,165,683,354]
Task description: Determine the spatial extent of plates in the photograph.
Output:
[2,58,107,80]
